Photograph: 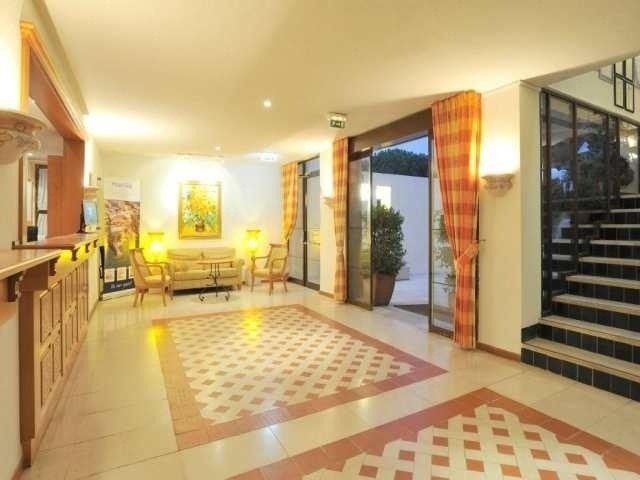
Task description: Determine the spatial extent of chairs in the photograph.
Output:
[129,249,174,307]
[250,238,291,297]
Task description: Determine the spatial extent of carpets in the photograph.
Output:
[394,304,449,324]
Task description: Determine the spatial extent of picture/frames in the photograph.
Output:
[599,57,640,112]
[178,180,222,240]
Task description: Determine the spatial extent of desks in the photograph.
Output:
[196,260,234,302]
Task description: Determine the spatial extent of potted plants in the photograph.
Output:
[355,198,408,307]
[434,210,456,317]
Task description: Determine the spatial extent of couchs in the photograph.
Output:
[168,247,245,300]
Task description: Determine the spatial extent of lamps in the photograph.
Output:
[482,175,512,197]
